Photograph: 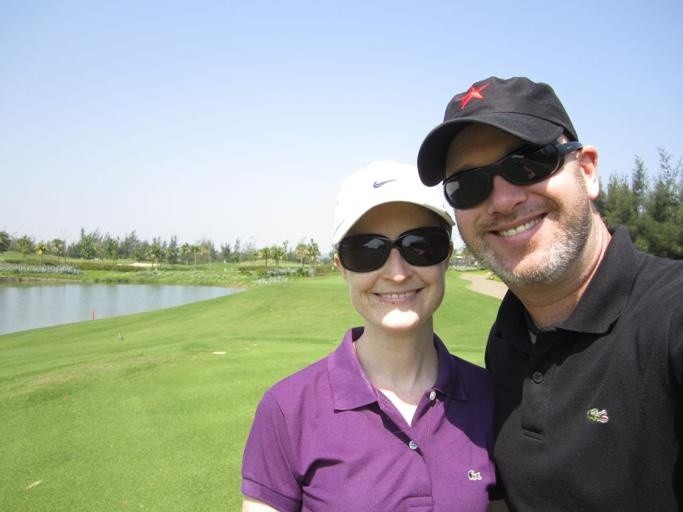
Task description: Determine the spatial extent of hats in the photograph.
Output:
[415,74,580,185]
[331,155,456,250]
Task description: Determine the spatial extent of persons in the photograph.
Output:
[416,78,681,511]
[241,162,503,512]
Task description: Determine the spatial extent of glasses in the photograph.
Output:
[336,226,451,275]
[440,137,582,208]
